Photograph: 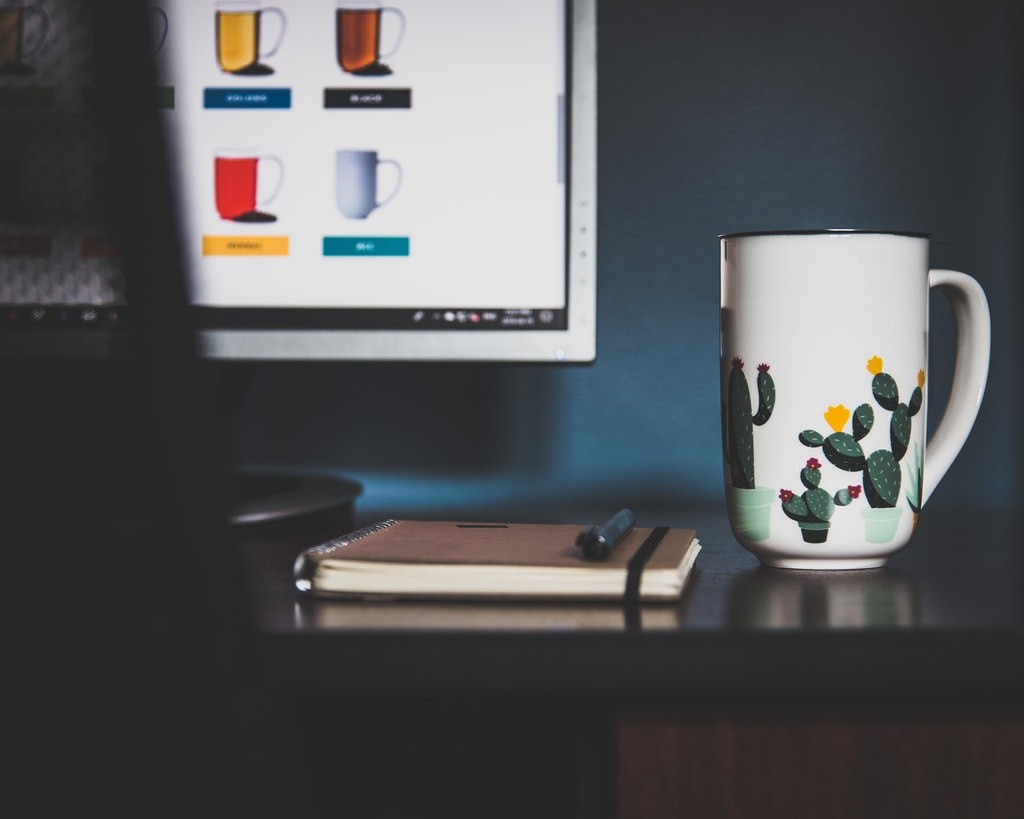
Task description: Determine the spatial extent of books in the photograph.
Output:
[296,519,704,603]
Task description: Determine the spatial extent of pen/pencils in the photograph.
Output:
[574,508,638,560]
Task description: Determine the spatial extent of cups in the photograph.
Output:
[210,0,404,220]
[718,229,993,570]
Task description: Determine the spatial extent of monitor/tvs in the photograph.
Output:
[0,0,601,365]
